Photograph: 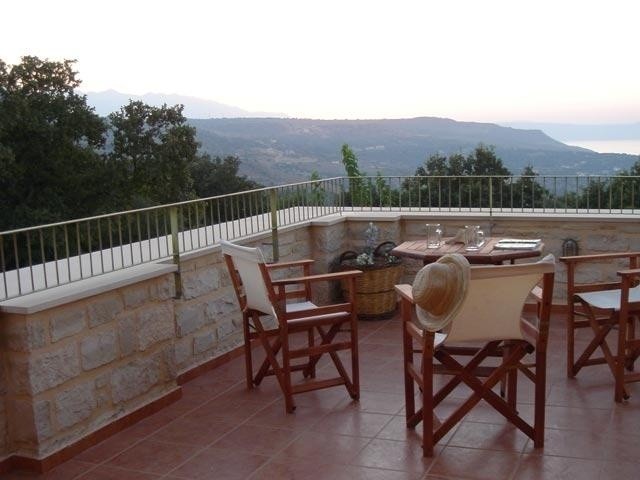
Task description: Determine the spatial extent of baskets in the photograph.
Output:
[341,242,403,320]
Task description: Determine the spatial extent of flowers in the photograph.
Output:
[356,222,380,264]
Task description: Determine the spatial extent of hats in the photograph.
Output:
[413,253,471,332]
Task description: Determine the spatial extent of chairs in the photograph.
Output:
[558,251,640,403]
[393,252,556,456]
[220,240,364,414]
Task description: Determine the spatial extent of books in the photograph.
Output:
[493,238,542,250]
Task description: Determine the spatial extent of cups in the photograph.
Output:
[465,225,484,251]
[426,223,443,248]
